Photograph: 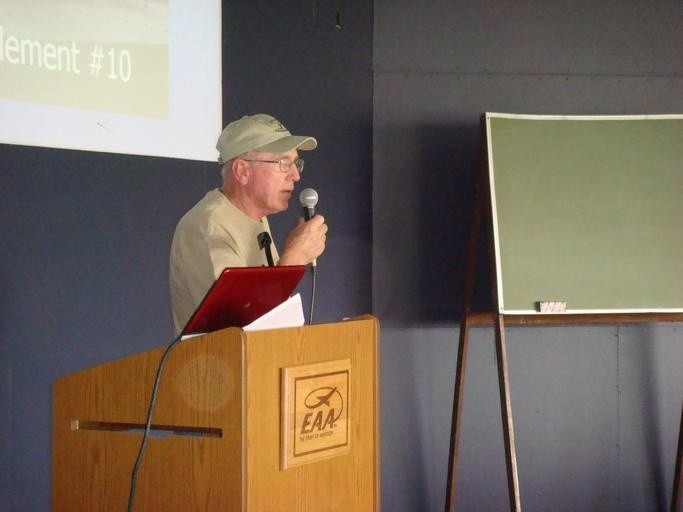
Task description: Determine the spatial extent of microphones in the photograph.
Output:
[299,188,319,272]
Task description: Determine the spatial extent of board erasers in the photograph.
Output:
[536,302,567,313]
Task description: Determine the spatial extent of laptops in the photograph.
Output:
[181,265,309,338]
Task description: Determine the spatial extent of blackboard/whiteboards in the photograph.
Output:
[483,111,682,316]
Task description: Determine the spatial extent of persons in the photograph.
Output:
[170,115,327,340]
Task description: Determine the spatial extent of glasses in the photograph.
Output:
[241,156,305,172]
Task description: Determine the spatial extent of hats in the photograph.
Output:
[216,113,317,163]
